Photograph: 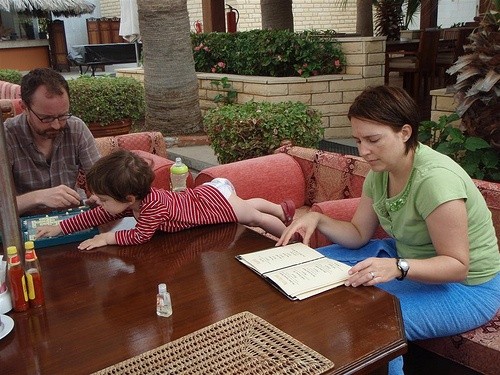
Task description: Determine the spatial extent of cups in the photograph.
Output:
[0,287,12,315]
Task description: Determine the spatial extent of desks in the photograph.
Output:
[0,223,409,375]
[386,39,456,95]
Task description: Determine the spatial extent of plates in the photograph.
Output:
[0,314,14,340]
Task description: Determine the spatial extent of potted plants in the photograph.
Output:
[66,74,144,138]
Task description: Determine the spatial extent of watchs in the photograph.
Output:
[396,257,410,281]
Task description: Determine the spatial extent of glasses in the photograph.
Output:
[26,105,72,122]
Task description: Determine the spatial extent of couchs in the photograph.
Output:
[195,146,500,375]
[78,131,194,198]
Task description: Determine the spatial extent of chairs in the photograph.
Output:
[385,27,440,96]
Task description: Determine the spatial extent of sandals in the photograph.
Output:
[281,200,299,242]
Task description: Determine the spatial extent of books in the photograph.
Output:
[235,242,359,302]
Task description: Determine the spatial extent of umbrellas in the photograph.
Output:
[119,0,141,68]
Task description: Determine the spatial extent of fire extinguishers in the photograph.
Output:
[195,20,202,33]
[225,3,237,33]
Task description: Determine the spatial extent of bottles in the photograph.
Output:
[156,284,172,318]
[7,246,29,312]
[24,241,45,309]
[169,157,189,192]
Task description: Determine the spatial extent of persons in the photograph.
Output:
[275,85,500,375]
[3,68,102,215]
[33,150,303,250]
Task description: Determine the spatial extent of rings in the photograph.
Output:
[369,271,375,279]
